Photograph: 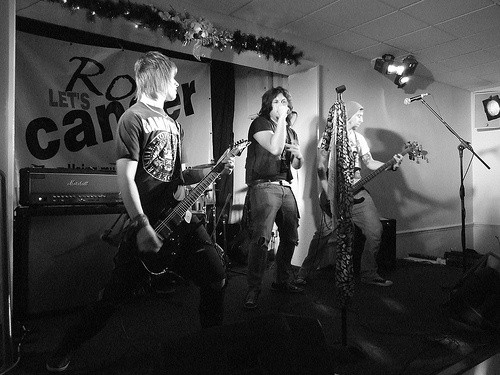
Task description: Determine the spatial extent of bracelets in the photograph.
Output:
[131,214,149,232]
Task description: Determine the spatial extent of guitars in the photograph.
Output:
[319,140,428,218]
[119,138,254,301]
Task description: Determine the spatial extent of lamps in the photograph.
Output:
[370,52,419,86]
[482,95,500,121]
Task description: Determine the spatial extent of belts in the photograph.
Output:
[250,179,291,187]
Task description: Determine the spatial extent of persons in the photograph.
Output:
[244,87,304,310]
[288,100,403,295]
[46,51,239,371]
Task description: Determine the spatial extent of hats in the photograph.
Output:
[344,101,362,121]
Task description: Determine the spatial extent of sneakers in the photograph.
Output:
[47,347,69,371]
[362,274,392,287]
[296,279,306,285]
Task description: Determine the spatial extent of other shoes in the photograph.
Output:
[271,281,304,292]
[246,294,257,305]
